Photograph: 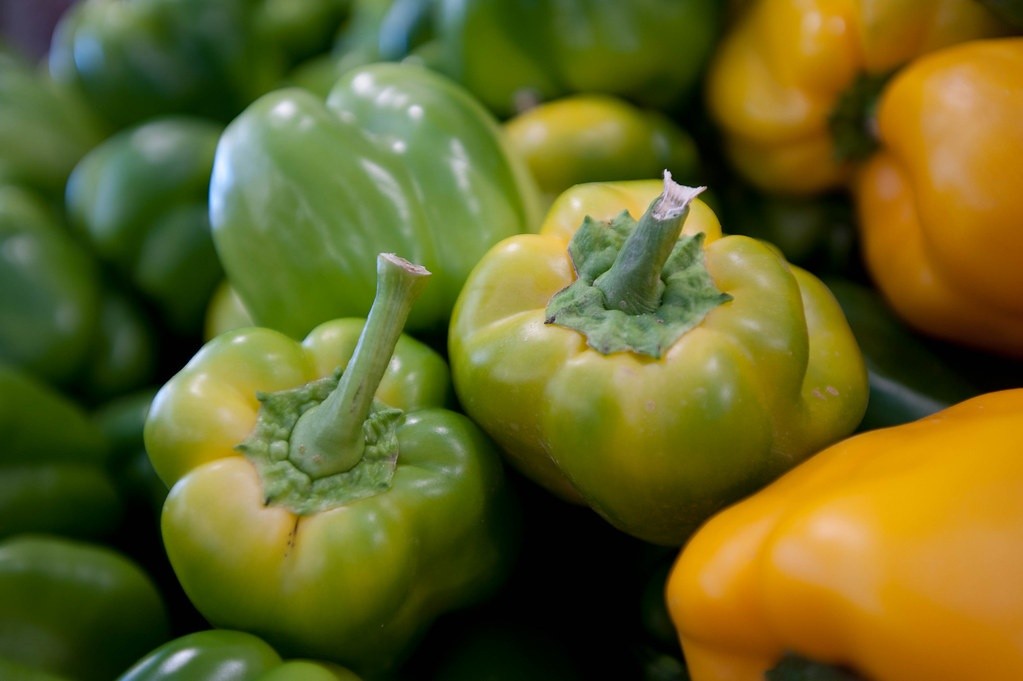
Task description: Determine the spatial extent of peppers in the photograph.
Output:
[0,0,1023,681]
